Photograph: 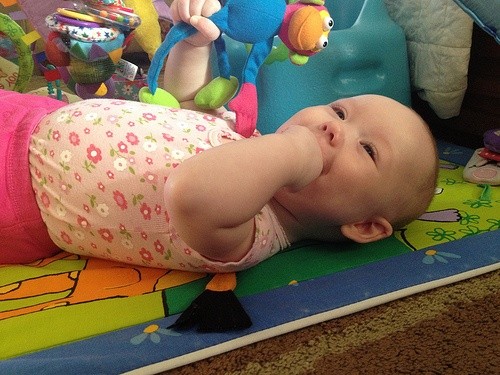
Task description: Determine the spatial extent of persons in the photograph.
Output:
[1,0,441,275]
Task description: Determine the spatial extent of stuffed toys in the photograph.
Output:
[138,0,333,139]
